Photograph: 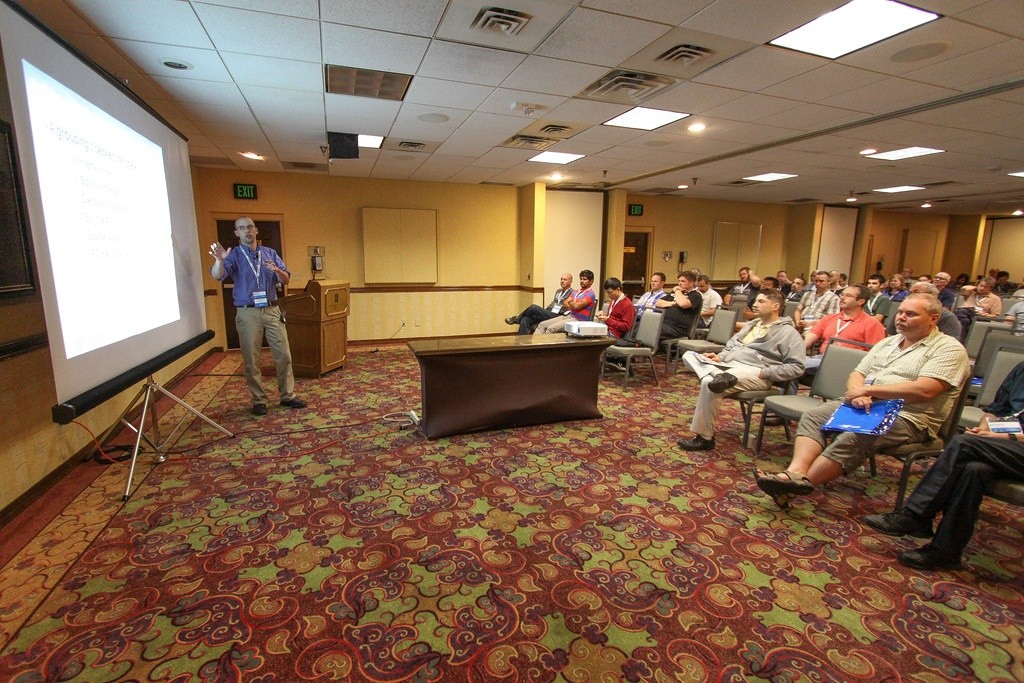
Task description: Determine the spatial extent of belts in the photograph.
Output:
[236,300,279,307]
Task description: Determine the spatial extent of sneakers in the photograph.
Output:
[898,542,962,571]
[864,506,935,538]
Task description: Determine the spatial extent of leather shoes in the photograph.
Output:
[707,371,738,393]
[676,435,715,450]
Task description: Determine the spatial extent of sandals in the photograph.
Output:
[752,467,790,509]
[757,470,814,496]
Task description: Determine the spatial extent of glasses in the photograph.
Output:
[236,224,254,230]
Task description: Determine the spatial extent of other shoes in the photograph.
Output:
[253,403,267,415]
[280,397,307,407]
[762,412,792,425]
[505,316,520,325]
[601,354,627,371]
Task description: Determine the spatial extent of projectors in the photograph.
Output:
[565,321,608,338]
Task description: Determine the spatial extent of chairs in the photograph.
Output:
[589,289,1024,516]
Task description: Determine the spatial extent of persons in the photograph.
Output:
[208,216,307,415]
[862,360,1024,569]
[634,265,1024,343]
[505,273,574,335]
[595,278,636,355]
[532,270,595,335]
[752,293,970,510]
[676,289,806,451]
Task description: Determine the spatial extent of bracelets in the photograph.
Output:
[1008,432,1017,441]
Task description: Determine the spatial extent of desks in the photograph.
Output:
[407,333,617,441]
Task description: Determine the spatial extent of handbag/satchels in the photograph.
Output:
[953,307,975,324]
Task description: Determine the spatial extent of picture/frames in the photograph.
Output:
[0,120,36,301]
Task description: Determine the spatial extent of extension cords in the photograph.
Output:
[409,411,420,426]
